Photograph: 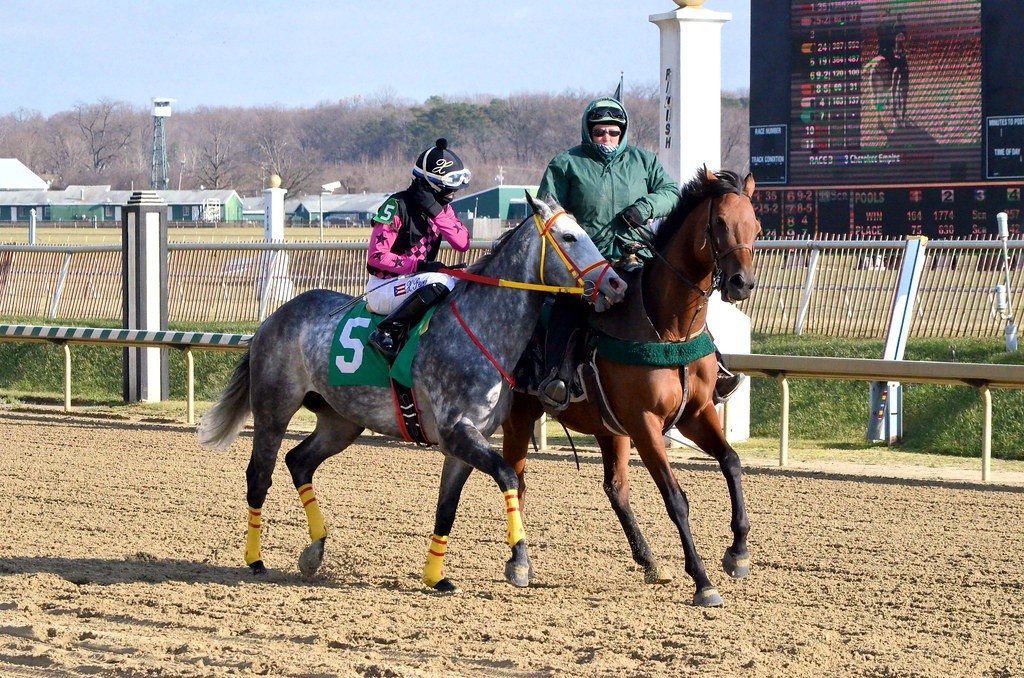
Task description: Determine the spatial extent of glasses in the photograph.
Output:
[589,106,626,120]
[423,146,459,197]
[442,169,472,187]
[590,129,621,137]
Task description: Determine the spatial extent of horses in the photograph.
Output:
[861,30,910,130]
[500,162,761,608]
[196,189,629,595]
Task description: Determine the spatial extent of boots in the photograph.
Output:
[711,372,746,405]
[538,299,581,414]
[368,284,445,359]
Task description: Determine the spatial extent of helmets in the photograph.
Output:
[410,138,470,189]
[586,98,628,125]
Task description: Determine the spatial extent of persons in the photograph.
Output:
[363,137,473,358]
[532,96,748,418]
[876,8,909,67]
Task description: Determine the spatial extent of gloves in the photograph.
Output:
[411,184,443,218]
[418,259,449,272]
[615,201,652,229]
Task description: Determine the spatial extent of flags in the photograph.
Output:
[612,78,623,104]
